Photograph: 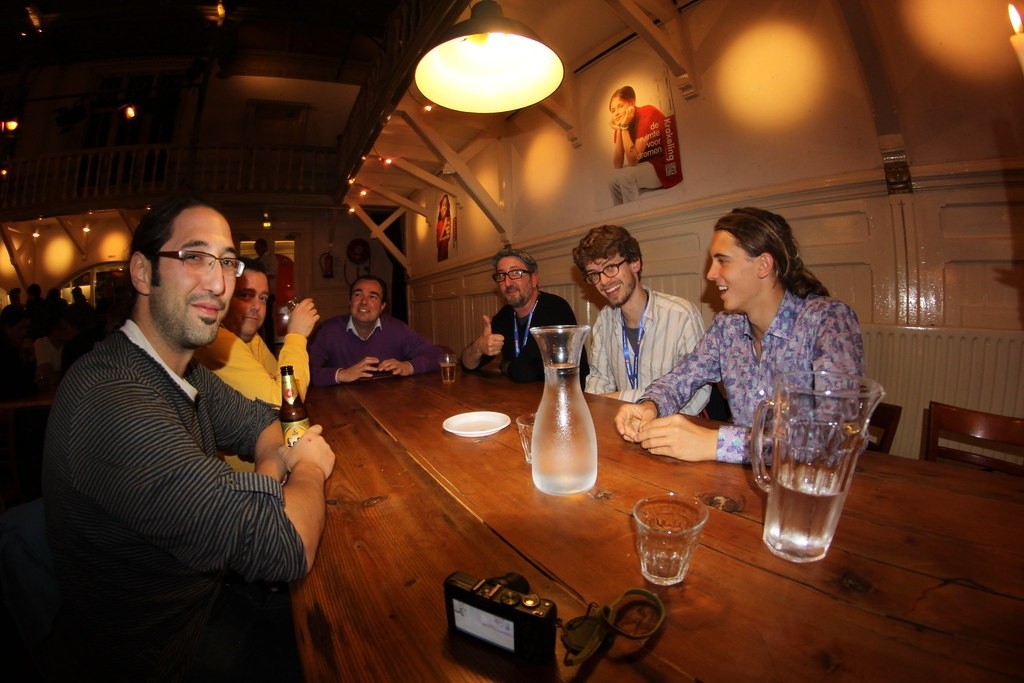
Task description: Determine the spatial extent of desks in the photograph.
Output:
[277,366,1024,683]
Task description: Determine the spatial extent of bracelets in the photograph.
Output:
[498,360,510,372]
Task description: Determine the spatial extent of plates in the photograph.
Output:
[442,411,511,437]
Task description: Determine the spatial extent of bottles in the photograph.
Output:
[530,324,598,494]
[279,365,309,447]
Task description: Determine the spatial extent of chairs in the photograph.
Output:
[926,399,1024,476]
[861,401,902,455]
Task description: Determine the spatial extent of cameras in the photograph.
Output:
[443,571,558,665]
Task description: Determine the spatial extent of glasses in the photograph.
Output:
[584,257,631,286]
[492,269,532,283]
[151,250,245,278]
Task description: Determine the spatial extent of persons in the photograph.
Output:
[0,306,37,505]
[61,296,119,378]
[39,288,68,317]
[21,198,336,683]
[64,287,94,326]
[615,207,868,467]
[436,194,451,263]
[308,276,450,386]
[26,283,44,309]
[31,313,70,376]
[0,288,25,316]
[571,223,712,415]
[608,85,683,206]
[461,248,590,382]
[192,257,320,485]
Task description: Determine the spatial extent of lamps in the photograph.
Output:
[412,0,566,113]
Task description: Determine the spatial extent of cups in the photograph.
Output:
[516,412,537,464]
[287,295,302,311]
[439,353,458,383]
[750,370,884,563]
[633,492,709,585]
